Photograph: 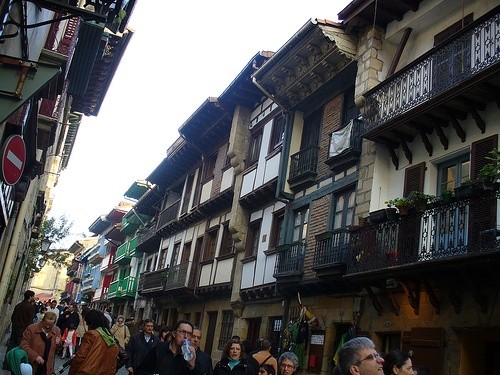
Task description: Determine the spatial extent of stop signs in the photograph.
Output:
[1,135,27,186]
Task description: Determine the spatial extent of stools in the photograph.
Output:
[479,229,500,252]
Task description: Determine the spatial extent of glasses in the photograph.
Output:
[178,329,191,337]
[354,353,382,366]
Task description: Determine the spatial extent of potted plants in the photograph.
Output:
[461,179,473,186]
[480,148,500,190]
[384,192,436,215]
[358,214,366,226]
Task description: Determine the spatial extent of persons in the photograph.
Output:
[20,312,64,375]
[214,339,259,375]
[29,299,174,360]
[192,325,214,375]
[337,336,385,375]
[134,320,204,375]
[258,364,275,375]
[125,319,161,375]
[242,339,260,369]
[382,351,414,375]
[2,290,38,371]
[251,339,277,375]
[232,335,240,340]
[68,309,129,375]
[278,352,299,375]
[250,337,265,356]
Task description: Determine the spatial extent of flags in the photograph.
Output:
[329,119,354,157]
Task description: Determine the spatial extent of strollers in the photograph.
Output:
[6,346,76,375]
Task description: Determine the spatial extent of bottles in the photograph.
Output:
[182,339,193,361]
